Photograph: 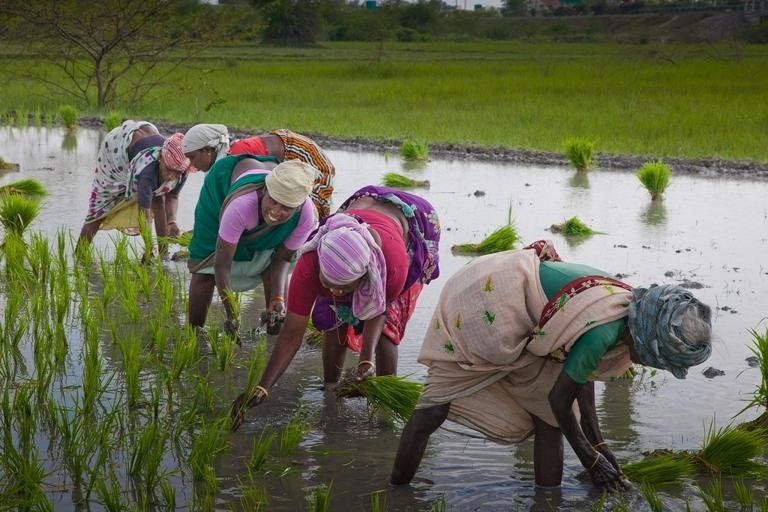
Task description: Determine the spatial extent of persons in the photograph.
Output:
[171,124,335,262]
[230,186,439,432]
[188,153,323,356]
[74,119,196,263]
[389,240,712,492]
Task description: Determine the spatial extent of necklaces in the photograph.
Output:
[332,295,353,347]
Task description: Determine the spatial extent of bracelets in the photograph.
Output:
[167,221,177,224]
[589,451,600,471]
[593,442,609,447]
[358,361,375,368]
[222,296,236,303]
[255,385,269,398]
[270,297,284,301]
